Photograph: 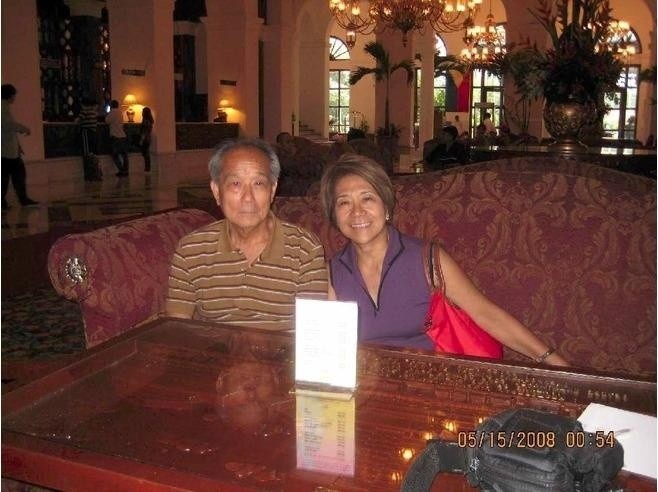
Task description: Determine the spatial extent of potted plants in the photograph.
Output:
[348,42,416,152]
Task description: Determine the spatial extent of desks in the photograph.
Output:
[0,316,656,492]
[471,145,657,176]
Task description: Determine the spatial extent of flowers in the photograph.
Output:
[505,0,622,123]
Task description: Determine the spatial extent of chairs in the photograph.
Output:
[423,133,554,169]
[177,137,392,207]
[637,133,654,150]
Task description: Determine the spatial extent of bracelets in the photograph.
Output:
[536,347,555,363]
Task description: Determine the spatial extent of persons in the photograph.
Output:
[139,107,154,172]
[105,100,132,175]
[75,100,102,178]
[166,140,328,334]
[424,125,465,167]
[2,84,40,207]
[277,132,310,184]
[452,113,497,153]
[321,154,572,367]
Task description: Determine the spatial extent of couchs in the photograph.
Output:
[46,149,657,380]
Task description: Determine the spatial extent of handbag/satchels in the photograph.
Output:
[423,291,503,360]
[401,408,624,492]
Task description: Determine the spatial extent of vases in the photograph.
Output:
[542,86,596,156]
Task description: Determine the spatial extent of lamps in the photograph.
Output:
[326,0,482,49]
[217,100,232,121]
[121,94,138,122]
[459,0,505,72]
[584,21,630,60]
